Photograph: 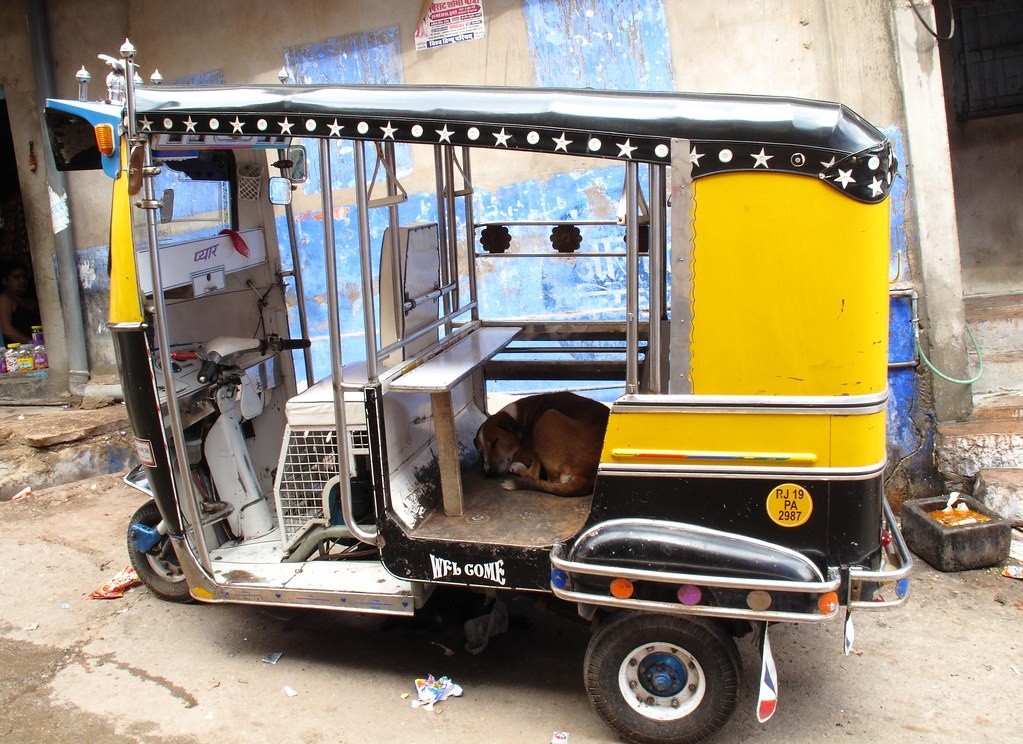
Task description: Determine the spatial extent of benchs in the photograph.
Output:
[284,220,440,432]
[387,326,523,518]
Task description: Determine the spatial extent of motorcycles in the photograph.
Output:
[44,37,915,744]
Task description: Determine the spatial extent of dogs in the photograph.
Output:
[474,391,610,498]
